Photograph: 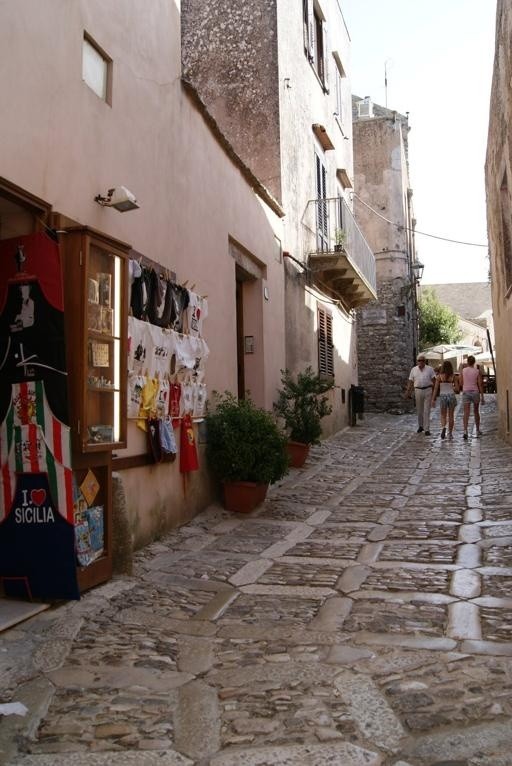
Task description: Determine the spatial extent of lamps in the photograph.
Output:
[92,185,140,212]
[401,257,424,300]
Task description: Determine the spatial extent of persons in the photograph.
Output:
[430,360,460,438]
[459,355,485,441]
[402,353,436,437]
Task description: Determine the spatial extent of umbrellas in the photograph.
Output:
[463,351,497,364]
[416,344,482,365]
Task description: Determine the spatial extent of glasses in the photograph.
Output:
[417,360,425,362]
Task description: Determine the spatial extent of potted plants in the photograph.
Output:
[273,367,334,467]
[334,227,344,251]
[207,389,288,512]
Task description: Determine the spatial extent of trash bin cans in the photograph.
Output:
[351,384,364,426]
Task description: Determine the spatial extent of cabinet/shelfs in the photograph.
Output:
[65,225,132,455]
[126,258,209,421]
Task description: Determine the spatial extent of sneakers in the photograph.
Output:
[417,426,424,433]
[447,433,453,439]
[441,426,446,439]
[464,429,468,439]
[425,431,430,436]
[475,432,482,438]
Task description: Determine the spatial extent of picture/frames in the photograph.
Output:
[245,335,254,353]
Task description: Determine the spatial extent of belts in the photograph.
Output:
[417,385,431,390]
